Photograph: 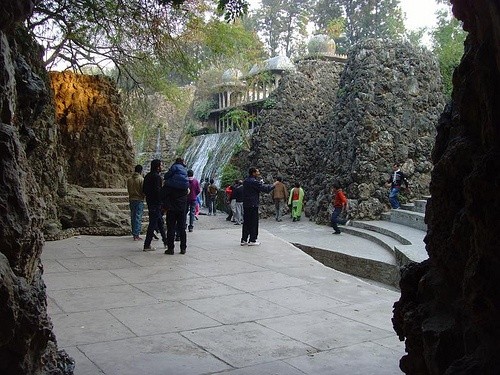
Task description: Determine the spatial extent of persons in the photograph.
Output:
[388,162,407,211]
[288,182,305,222]
[127,157,219,255]
[225,180,244,225]
[273,177,288,222]
[331,182,348,234]
[241,167,279,246]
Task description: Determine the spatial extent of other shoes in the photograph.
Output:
[297,218,301,221]
[234,222,241,224]
[333,232,342,235]
[345,219,351,226]
[249,241,262,246]
[241,240,248,245]
[152,236,159,241]
[143,246,156,251]
[134,236,140,240]
[176,237,181,240]
[165,250,173,253]
[293,218,297,222]
[276,218,282,222]
[180,250,185,253]
[189,227,193,231]
